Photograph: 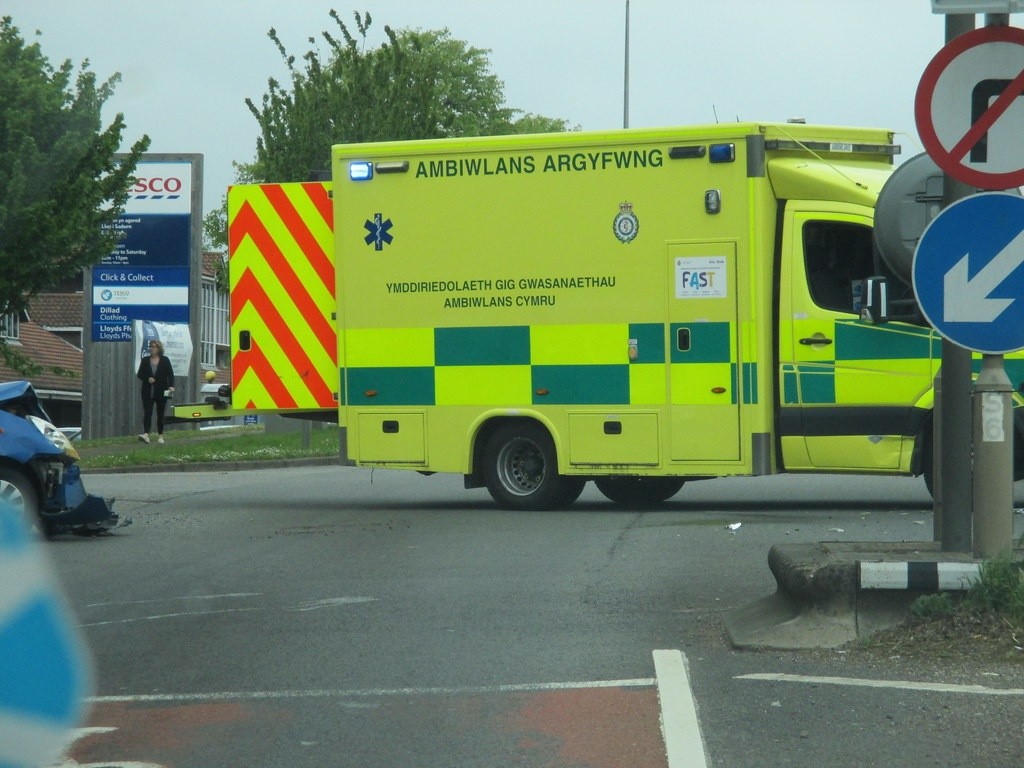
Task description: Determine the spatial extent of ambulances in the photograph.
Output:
[172,117,1023,516]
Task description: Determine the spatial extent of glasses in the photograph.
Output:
[148,345,158,349]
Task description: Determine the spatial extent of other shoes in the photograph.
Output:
[158,438,164,444]
[138,435,149,444]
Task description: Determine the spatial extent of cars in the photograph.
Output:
[0,380,133,537]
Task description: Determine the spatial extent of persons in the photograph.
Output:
[137,340,175,443]
